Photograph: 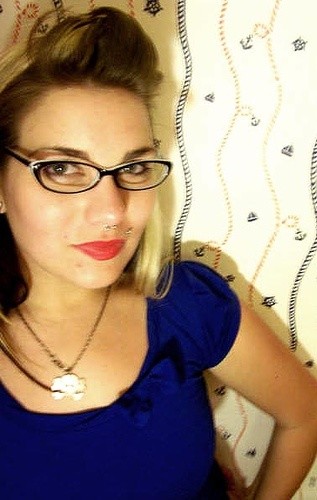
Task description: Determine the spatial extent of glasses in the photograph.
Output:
[0,145,173,193]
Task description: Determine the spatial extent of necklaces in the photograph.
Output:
[15,286,112,400]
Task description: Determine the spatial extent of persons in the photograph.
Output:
[1,7,317,500]
